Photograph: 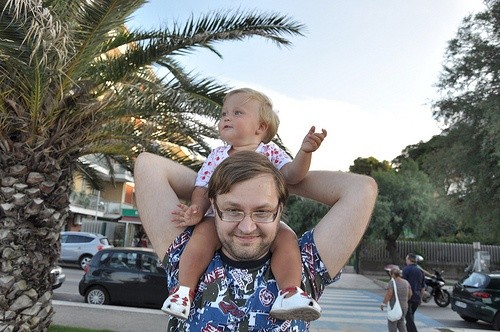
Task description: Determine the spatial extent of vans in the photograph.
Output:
[59,231,115,270]
[79,247,171,306]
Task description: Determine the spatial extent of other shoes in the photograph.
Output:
[161,285,195,320]
[267,287,322,322]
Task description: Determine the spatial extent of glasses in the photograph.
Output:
[213,201,282,222]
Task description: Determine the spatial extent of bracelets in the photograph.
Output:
[381,303,386,306]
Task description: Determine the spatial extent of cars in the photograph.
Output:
[49,264,66,292]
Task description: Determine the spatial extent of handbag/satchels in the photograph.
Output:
[384,278,402,322]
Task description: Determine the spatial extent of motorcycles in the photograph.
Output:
[422,266,450,307]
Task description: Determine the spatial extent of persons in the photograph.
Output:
[380,266,413,332]
[134,152,378,332]
[401,253,425,332]
[162,88,328,321]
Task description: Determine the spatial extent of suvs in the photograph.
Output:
[451,271,500,329]
